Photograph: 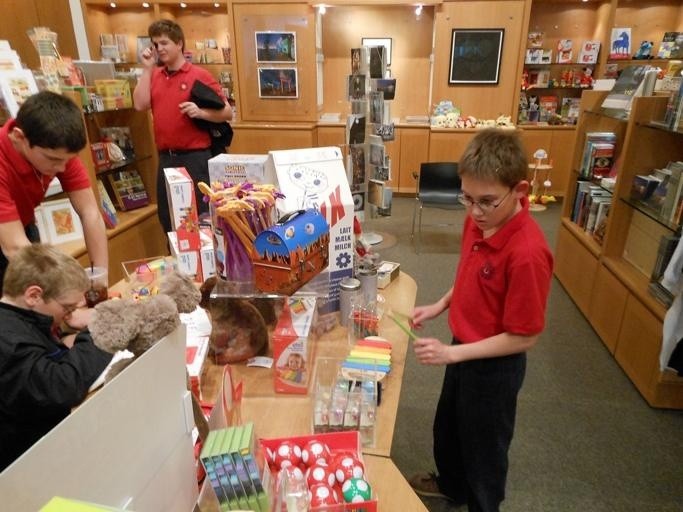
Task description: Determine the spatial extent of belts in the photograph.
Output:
[159,148,205,157]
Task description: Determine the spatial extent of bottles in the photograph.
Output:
[338,277,362,326]
[201,53,208,64]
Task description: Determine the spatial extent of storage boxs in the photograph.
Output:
[164,146,355,396]
[622,209,676,278]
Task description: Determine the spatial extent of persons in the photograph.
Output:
[0,90,108,339]
[408,128,554,511]
[283,352,305,370]
[0,242,114,469]
[133,19,233,256]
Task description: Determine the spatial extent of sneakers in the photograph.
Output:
[411,471,461,502]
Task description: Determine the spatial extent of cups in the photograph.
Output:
[222,48,232,65]
[357,268,378,308]
[80,266,108,307]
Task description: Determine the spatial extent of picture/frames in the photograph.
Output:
[40,197,86,246]
[30,207,47,247]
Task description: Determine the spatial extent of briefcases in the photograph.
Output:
[251,208,329,296]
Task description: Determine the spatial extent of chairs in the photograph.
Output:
[410,160,469,246]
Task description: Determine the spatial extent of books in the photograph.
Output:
[96,174,119,229]
[0,39,84,244]
[346,46,395,223]
[405,115,429,126]
[319,112,342,124]
[570,61,682,307]
[108,170,151,211]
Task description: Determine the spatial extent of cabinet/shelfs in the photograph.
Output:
[0,1,682,201]
[0,85,159,303]
[553,89,682,411]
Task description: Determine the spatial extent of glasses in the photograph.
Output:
[456,180,521,209]
[48,293,81,314]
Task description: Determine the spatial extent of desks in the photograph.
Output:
[105,244,422,461]
[193,453,430,510]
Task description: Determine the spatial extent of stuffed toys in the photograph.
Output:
[198,275,266,365]
[86,269,202,383]
[431,100,515,129]
